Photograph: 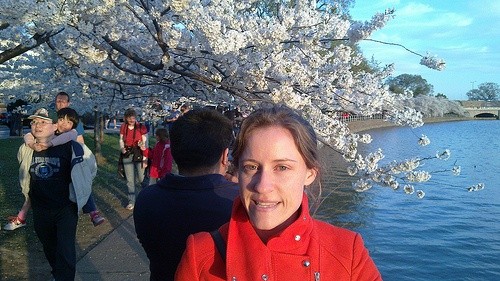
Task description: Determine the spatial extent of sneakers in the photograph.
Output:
[3,216,26,231]
[90,212,104,228]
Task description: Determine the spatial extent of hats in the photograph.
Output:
[27,107,58,122]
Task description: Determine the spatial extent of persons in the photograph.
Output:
[174,105,383,281]
[16,106,99,281]
[117,107,149,209]
[55,92,84,143]
[4,107,104,230]
[138,128,172,182]
[141,99,243,172]
[134,107,241,281]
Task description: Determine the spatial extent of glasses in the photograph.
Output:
[29,120,54,126]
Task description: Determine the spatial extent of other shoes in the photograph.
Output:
[125,204,134,210]
